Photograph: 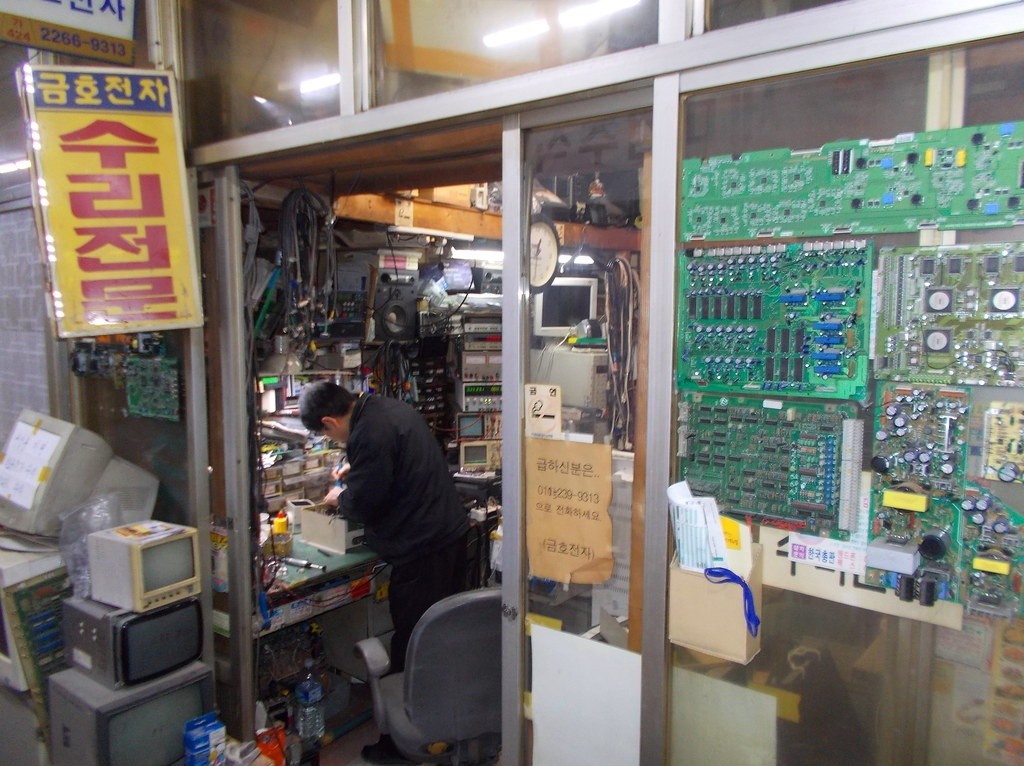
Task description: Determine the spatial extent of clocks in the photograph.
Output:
[530,213,560,294]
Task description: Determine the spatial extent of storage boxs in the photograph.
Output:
[181,711,226,766]
[262,449,342,514]
[418,184,471,208]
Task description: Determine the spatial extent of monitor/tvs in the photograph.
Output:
[463,444,487,466]
[87,519,202,613]
[533,276,598,351]
[441,259,476,296]
[62,597,205,691]
[51,667,216,766]
[0,408,159,552]
[457,414,486,439]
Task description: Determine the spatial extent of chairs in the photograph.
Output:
[353,585,501,766]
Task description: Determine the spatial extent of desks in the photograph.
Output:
[213,531,388,745]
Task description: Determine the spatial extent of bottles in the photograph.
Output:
[295,658,325,739]
[569,319,605,338]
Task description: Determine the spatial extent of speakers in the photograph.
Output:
[372,269,416,341]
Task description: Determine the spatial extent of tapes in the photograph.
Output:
[266,532,293,556]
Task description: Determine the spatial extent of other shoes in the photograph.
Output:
[361,734,416,766]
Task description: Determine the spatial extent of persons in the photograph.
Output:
[298,381,502,765]
